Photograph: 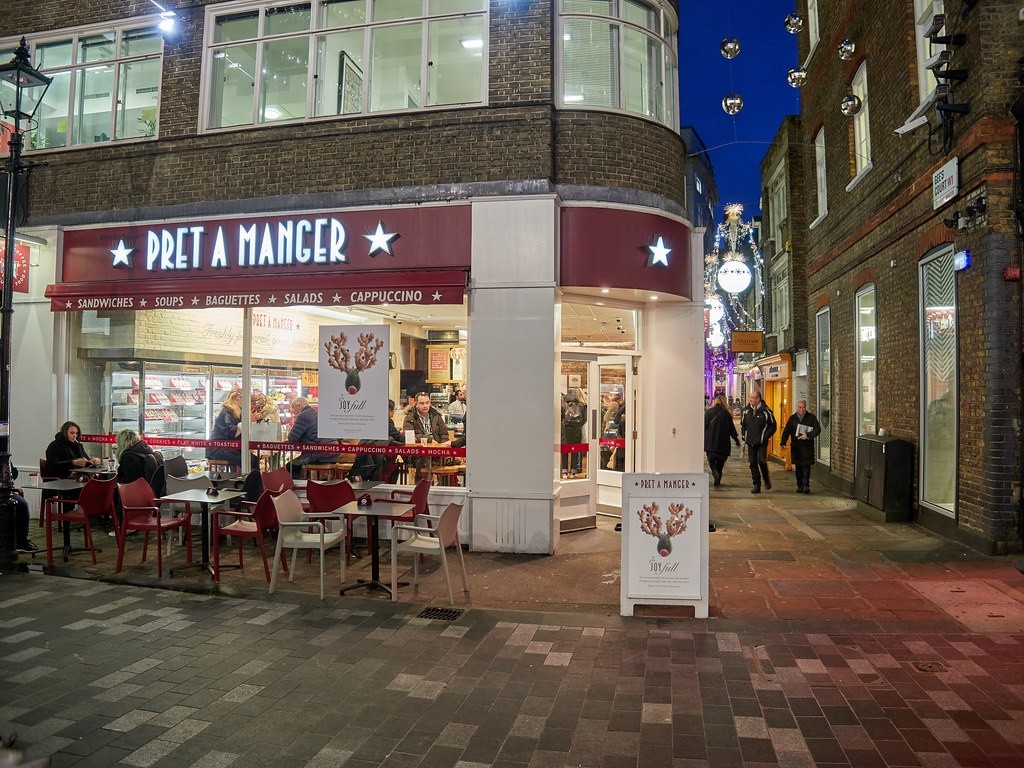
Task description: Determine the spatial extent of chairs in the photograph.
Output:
[14,455,470,607]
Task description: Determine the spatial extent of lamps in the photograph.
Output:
[158,11,196,33]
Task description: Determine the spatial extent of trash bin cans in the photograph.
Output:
[853,431,916,509]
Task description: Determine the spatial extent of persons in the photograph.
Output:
[45,422,155,536]
[560,388,626,480]
[11,460,40,553]
[208,386,466,480]
[704,388,821,492]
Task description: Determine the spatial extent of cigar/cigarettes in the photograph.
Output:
[74,439,78,443]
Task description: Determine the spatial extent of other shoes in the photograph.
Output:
[751,486,761,493]
[803,486,811,494]
[569,469,582,474]
[765,481,771,490]
[714,481,720,486]
[796,487,803,493]
[108,528,135,536]
[562,469,567,475]
[712,470,719,481]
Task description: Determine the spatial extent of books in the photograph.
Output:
[796,424,813,440]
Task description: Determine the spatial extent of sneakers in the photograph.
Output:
[16,540,38,553]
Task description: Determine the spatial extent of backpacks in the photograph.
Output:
[564,403,583,427]
[124,451,166,498]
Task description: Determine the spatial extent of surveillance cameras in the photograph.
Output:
[892,116,928,137]
[922,12,946,37]
[927,83,949,102]
[924,49,953,69]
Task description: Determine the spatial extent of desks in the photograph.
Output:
[21,479,103,561]
[72,467,118,475]
[161,488,247,580]
[405,443,451,485]
[333,501,416,600]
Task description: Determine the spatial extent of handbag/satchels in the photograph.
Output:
[607,447,619,469]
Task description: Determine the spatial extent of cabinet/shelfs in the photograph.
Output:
[101,361,302,475]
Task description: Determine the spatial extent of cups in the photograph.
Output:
[421,438,427,445]
[405,430,415,444]
[108,460,115,472]
[29,473,39,487]
[103,457,109,466]
[448,430,454,441]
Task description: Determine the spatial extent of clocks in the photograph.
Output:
[389,352,397,369]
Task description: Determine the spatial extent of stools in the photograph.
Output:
[208,460,233,473]
[302,464,338,482]
[433,466,459,487]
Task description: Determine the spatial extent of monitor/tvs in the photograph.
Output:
[400,369,425,388]
